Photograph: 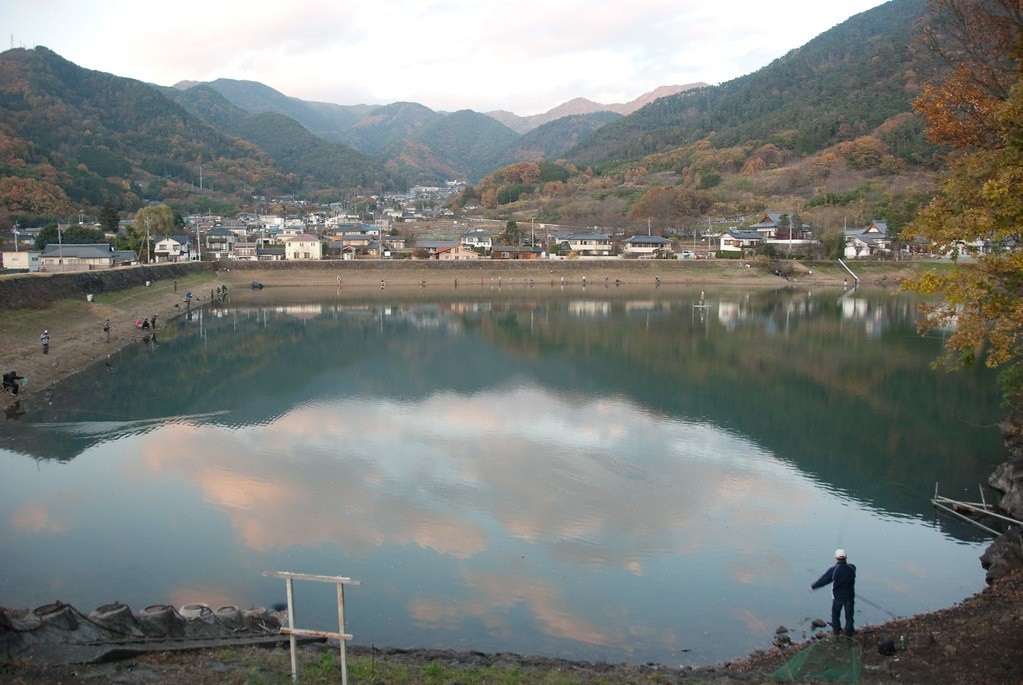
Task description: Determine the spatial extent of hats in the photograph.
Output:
[45,330,48,334]
[835,549,847,559]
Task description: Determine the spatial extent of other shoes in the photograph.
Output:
[10,392,16,396]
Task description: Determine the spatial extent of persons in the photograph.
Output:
[185,291,192,311]
[7,371,25,397]
[808,549,859,642]
[104,318,111,344]
[337,274,343,285]
[210,283,228,303]
[150,313,159,329]
[143,318,150,331]
[560,276,661,283]
[40,330,50,355]
